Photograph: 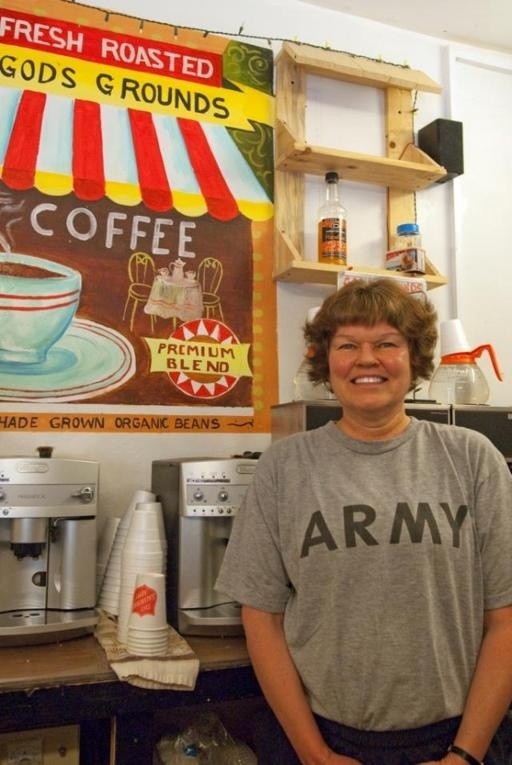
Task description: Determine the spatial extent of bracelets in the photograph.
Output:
[447,745,481,765]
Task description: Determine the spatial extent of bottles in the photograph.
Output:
[292,351,338,401]
[316,170,349,267]
[392,223,425,277]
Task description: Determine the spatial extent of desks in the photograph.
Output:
[0,631,282,765]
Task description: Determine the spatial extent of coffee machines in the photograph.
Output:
[0,451,102,649]
[149,456,261,638]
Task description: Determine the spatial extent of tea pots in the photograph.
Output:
[427,343,504,406]
[168,258,187,281]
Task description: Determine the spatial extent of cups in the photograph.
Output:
[0,250,83,366]
[94,488,171,658]
[437,319,472,357]
[158,268,169,279]
[186,271,196,282]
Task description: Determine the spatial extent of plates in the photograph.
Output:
[0,315,139,405]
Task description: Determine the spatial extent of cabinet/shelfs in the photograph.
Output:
[274,40,449,290]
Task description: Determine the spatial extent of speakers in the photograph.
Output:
[417,117,464,183]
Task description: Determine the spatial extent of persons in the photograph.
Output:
[214,277,511,765]
